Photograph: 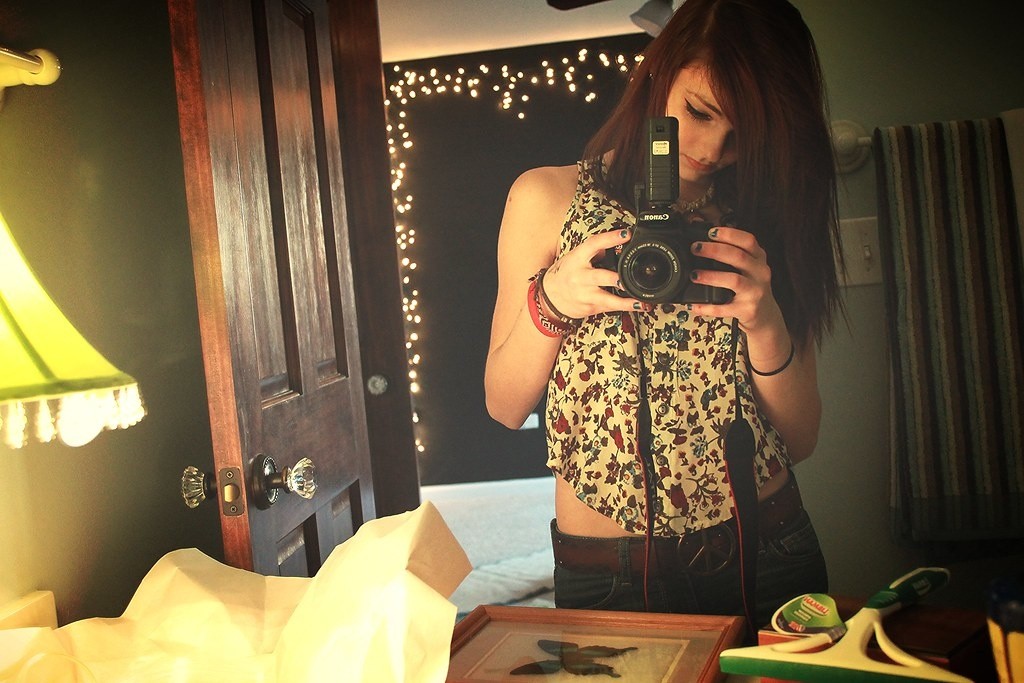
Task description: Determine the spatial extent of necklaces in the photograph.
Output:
[675,178,716,213]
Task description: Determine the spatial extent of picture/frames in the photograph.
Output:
[445,604,746,683]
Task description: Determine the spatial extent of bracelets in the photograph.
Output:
[527,268,584,337]
[750,342,794,376]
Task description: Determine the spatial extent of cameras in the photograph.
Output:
[603,118,737,305]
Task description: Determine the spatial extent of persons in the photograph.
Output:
[484,0,855,651]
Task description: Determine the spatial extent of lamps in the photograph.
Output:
[0,48,147,448]
